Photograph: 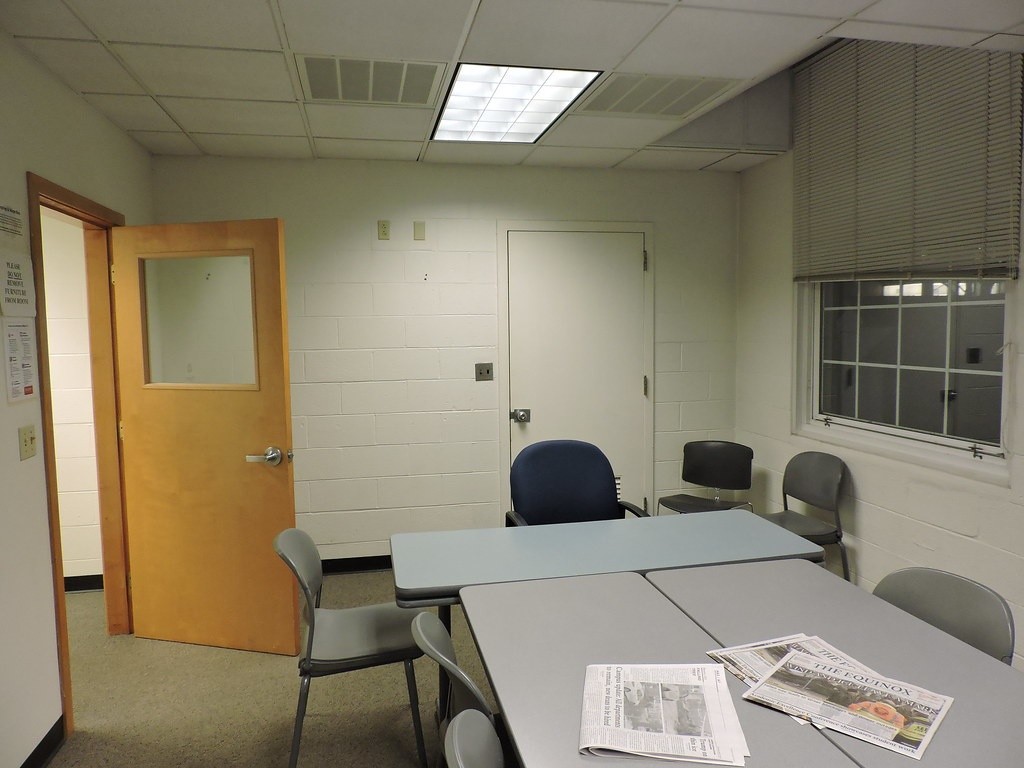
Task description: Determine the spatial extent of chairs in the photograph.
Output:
[657,441,753,515]
[872,566,1014,666]
[760,452,850,581]
[273,528,429,768]
[505,440,650,528]
[411,611,503,768]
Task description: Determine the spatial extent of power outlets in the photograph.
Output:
[378,220,391,240]
[18,424,37,462]
[475,362,493,381]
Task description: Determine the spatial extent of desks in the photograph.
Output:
[390,509,1024,768]
